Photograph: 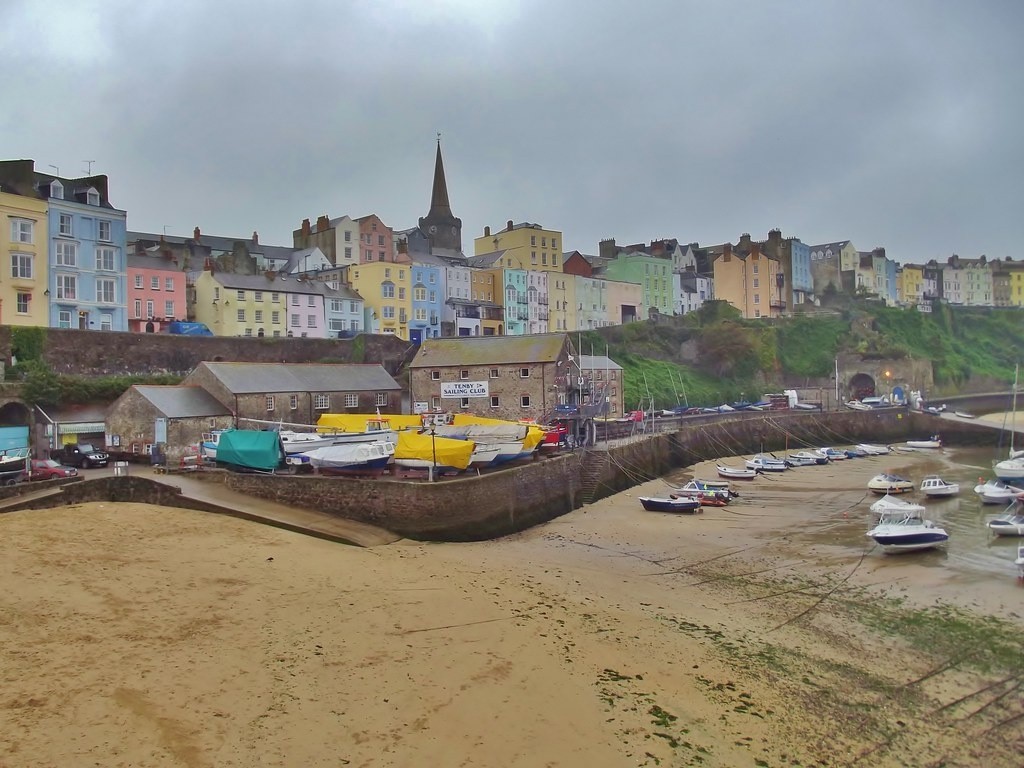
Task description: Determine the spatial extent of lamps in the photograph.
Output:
[225,300,229,305]
[44,289,50,296]
[212,300,217,305]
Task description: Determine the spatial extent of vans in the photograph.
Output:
[168,321,213,336]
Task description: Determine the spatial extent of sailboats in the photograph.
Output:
[988,362,1024,484]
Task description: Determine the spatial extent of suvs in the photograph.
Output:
[63,442,111,469]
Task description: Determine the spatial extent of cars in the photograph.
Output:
[31,459,79,479]
[624,410,644,422]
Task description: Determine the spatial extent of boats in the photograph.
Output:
[920,474,962,497]
[202,408,569,477]
[920,408,941,417]
[891,446,914,453]
[863,508,950,551]
[1015,546,1024,580]
[905,435,942,448]
[954,410,976,418]
[647,395,819,418]
[973,476,1024,504]
[867,496,929,522]
[623,436,895,507]
[639,497,702,513]
[843,399,877,412]
[865,473,916,496]
[984,497,1024,536]
